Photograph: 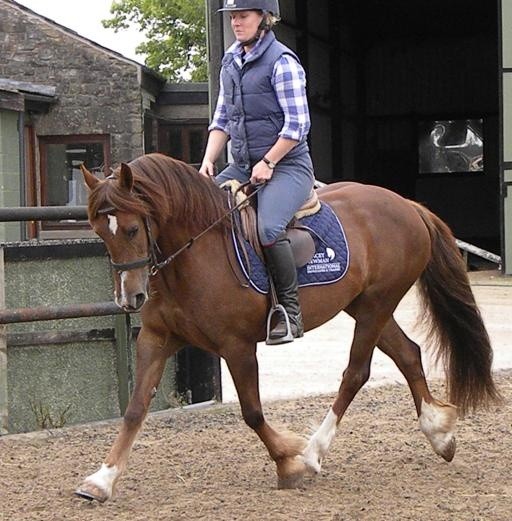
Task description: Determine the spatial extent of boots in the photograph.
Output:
[260,239,304,338]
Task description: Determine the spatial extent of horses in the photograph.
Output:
[72,152,507,504]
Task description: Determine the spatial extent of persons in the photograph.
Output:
[197,0,317,340]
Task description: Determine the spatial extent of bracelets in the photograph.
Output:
[261,157,276,169]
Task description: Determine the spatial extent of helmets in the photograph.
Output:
[216,0,280,15]
